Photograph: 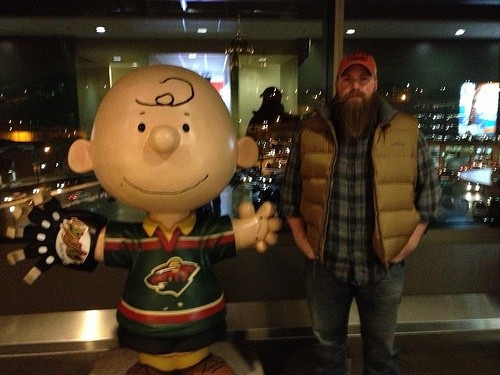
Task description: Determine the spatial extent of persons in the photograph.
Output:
[242,86,287,222]
[275,53,436,375]
[6,64,282,375]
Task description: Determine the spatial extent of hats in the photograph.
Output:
[339,51,377,78]
